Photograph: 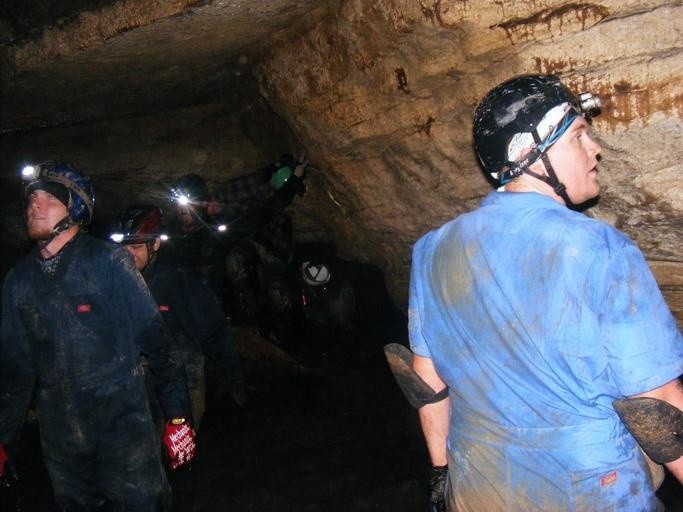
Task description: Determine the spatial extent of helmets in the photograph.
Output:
[114,202,163,274]
[20,157,95,251]
[170,173,209,226]
[470,73,601,212]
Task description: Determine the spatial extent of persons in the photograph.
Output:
[408,75,682,510]
[2,142,409,507]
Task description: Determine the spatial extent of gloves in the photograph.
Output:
[0,442,18,487]
[429,464,449,512]
[163,417,197,471]
